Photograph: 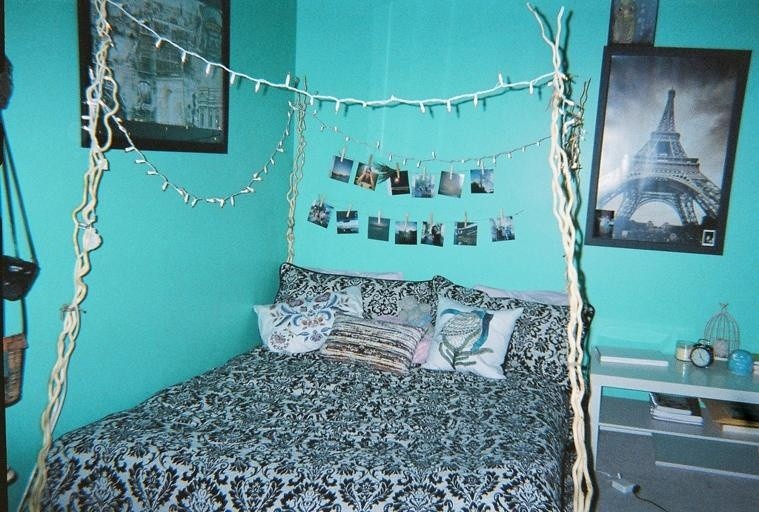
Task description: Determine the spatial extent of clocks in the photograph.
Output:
[690,343,714,368]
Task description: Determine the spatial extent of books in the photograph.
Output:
[646,389,703,426]
[703,398,759,436]
[592,345,674,367]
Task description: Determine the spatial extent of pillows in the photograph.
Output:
[318,312,427,376]
[274,262,436,362]
[432,275,595,385]
[423,296,523,381]
[252,284,364,354]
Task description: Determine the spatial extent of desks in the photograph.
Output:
[584,348,758,480]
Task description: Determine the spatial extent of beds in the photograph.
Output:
[35,308,586,512]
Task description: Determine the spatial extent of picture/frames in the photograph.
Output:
[78,0,231,155]
[582,43,756,254]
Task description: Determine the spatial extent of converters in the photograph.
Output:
[611,478,640,493]
